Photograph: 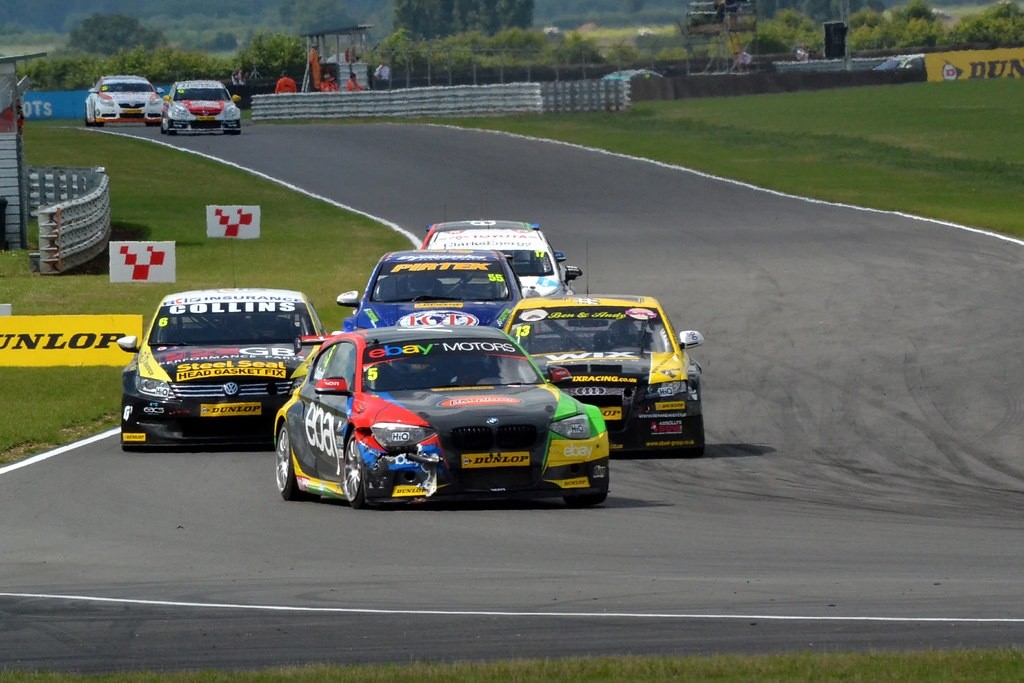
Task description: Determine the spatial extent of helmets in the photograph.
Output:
[249,313,279,337]
[606,319,639,348]
[407,272,435,297]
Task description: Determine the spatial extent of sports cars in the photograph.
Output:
[491,294,706,458]
[84,74,165,127]
[273,324,610,509]
[418,220,582,318]
[159,80,241,135]
[115,287,346,452]
[336,250,542,372]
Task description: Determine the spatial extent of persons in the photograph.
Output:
[231,67,248,98]
[374,59,390,90]
[593,319,640,352]
[346,73,363,91]
[320,73,338,92]
[275,71,297,94]
